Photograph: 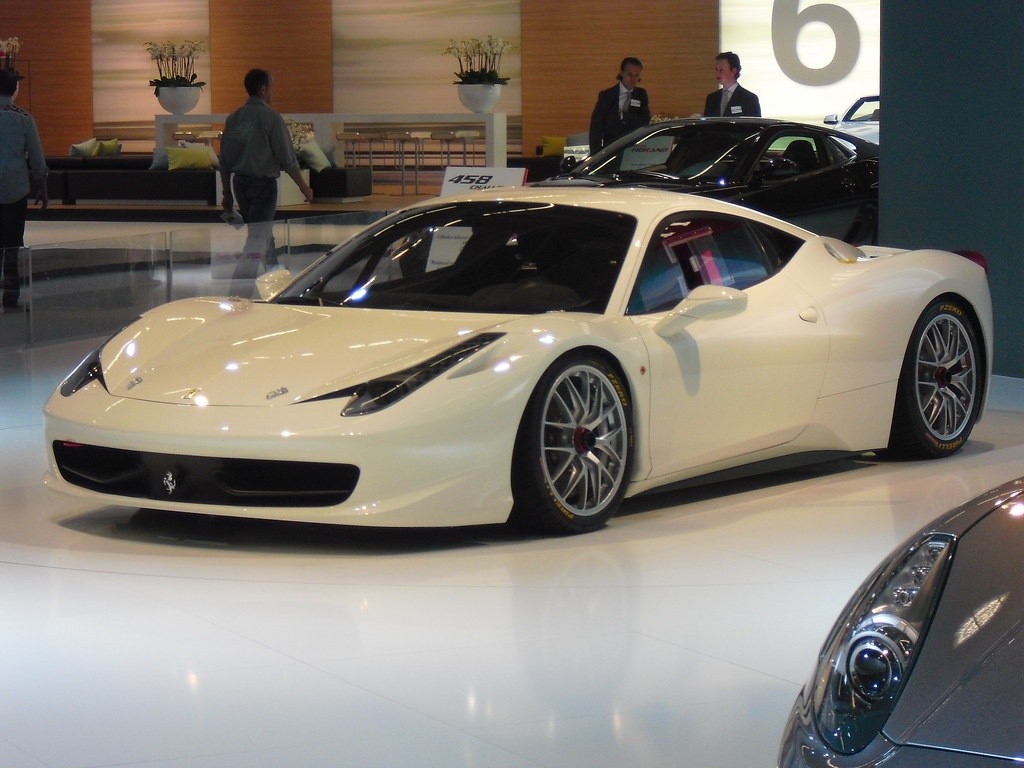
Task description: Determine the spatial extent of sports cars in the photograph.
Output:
[39,183,994,538]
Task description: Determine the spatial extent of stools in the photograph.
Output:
[335,127,480,193]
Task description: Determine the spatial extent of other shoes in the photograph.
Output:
[4,304,30,314]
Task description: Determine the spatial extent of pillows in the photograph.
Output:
[180,141,219,166]
[297,139,332,173]
[148,144,179,170]
[69,138,97,156]
[91,137,120,157]
[541,136,568,157]
[164,146,215,171]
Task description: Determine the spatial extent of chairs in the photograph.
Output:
[780,139,818,173]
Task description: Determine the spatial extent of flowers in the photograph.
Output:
[0,36,24,71]
[440,34,513,87]
[143,39,207,98]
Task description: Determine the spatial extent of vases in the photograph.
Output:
[457,83,502,114]
[156,87,201,114]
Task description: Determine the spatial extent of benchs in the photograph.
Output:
[334,165,371,197]
[23,155,217,206]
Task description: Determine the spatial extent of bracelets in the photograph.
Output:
[222,190,231,195]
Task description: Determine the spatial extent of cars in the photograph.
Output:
[822,96,884,153]
[525,116,879,246]
[773,476,1023,768]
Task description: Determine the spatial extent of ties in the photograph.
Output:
[621,92,631,119]
[721,90,730,117]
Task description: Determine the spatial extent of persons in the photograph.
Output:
[0,70,49,311]
[221,69,314,298]
[704,52,761,118]
[589,57,651,158]
